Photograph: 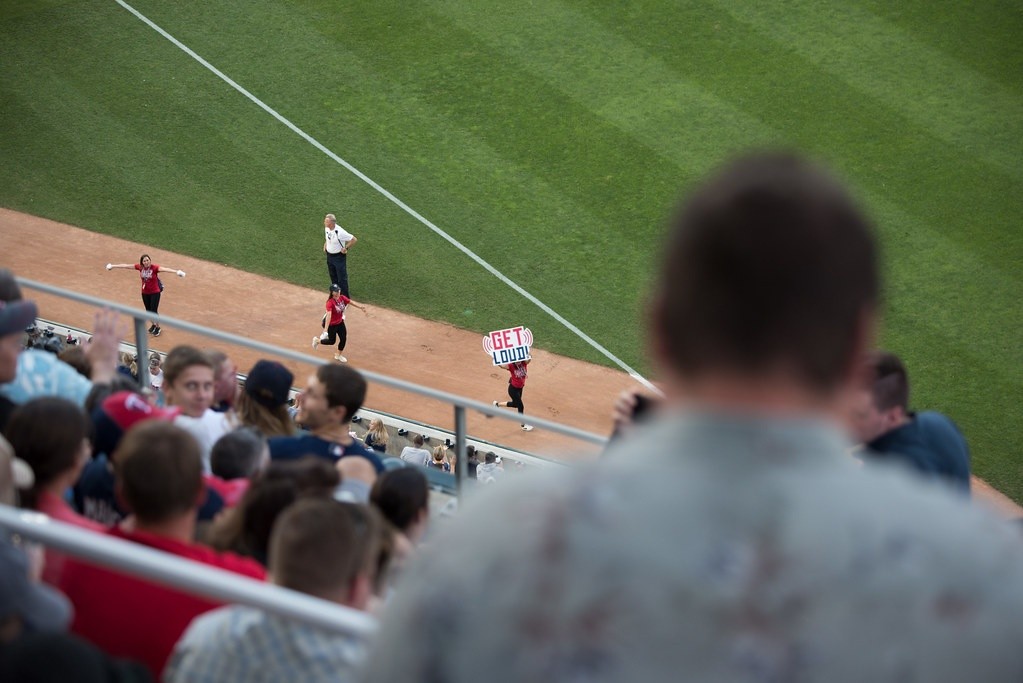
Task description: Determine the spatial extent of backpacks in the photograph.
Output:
[140,265,164,292]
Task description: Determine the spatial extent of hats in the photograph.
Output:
[329,284,341,292]
[86,389,188,462]
[0,298,38,337]
[245,359,294,410]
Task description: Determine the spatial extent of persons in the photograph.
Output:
[311,283,367,363]
[492,353,534,432]
[362,416,389,454]
[106,254,186,337]
[400,433,431,467]
[0,539,158,683]
[159,497,380,683]
[57,422,267,682]
[345,140,1022,683]
[465,443,481,479]
[0,265,434,600]
[475,448,505,483]
[427,443,457,475]
[321,213,357,299]
[847,348,972,502]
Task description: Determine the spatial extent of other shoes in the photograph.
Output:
[492,401,497,407]
[153,327,161,336]
[334,353,347,362]
[148,325,156,333]
[520,423,534,431]
[312,337,319,350]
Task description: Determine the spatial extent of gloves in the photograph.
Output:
[176,270,185,277]
[105,263,112,270]
